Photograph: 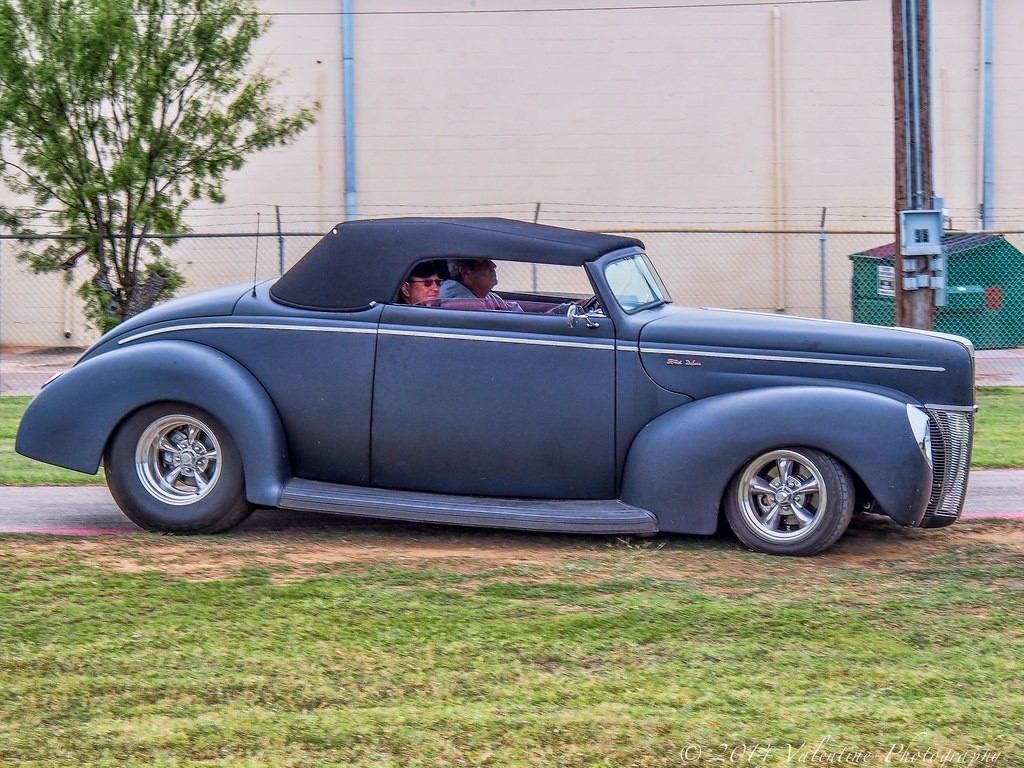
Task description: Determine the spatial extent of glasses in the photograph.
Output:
[409,279,443,287]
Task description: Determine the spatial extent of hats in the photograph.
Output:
[408,261,440,278]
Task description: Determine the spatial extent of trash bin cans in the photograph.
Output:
[847,233,1024,353]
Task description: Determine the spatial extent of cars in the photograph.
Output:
[14,216,979,557]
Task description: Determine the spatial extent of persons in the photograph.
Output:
[399,258,599,315]
